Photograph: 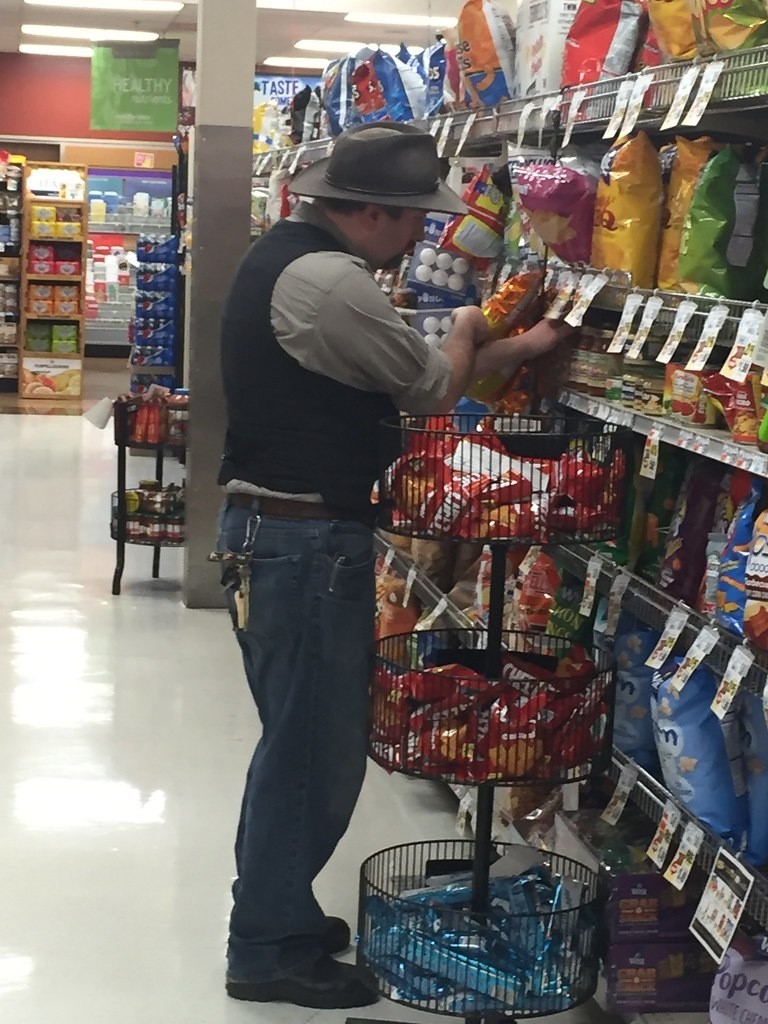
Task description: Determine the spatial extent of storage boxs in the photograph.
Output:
[27,324,52,339]
[28,285,53,301]
[31,222,55,237]
[52,326,77,341]
[54,286,80,302]
[29,245,53,260]
[56,222,81,238]
[26,338,52,352]
[27,261,55,275]
[31,206,56,222]
[52,340,77,354]
[55,262,81,275]
[53,301,78,314]
[27,300,53,314]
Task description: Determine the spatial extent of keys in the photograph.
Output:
[233,565,250,628]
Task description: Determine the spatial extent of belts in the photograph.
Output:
[228,492,375,521]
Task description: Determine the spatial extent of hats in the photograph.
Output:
[287,121,470,216]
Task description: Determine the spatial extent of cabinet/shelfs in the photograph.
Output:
[0,0,768,1024]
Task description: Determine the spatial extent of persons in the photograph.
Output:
[209,121,602,1009]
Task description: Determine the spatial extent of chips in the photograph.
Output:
[371,698,545,776]
[401,475,517,538]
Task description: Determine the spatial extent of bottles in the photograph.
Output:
[167,389,188,437]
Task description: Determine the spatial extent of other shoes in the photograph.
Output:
[318,915,350,955]
[225,950,380,1009]
